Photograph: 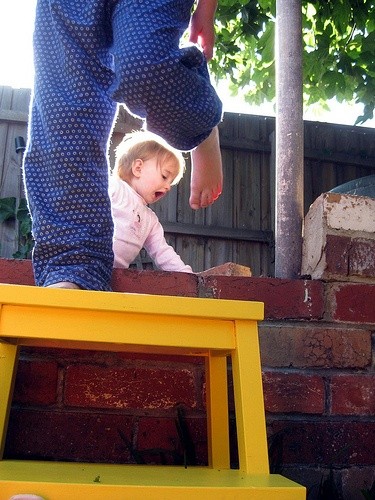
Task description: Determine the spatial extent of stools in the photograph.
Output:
[0,282,306,500]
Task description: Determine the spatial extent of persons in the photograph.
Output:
[24,0,225,293]
[106,128,194,274]
